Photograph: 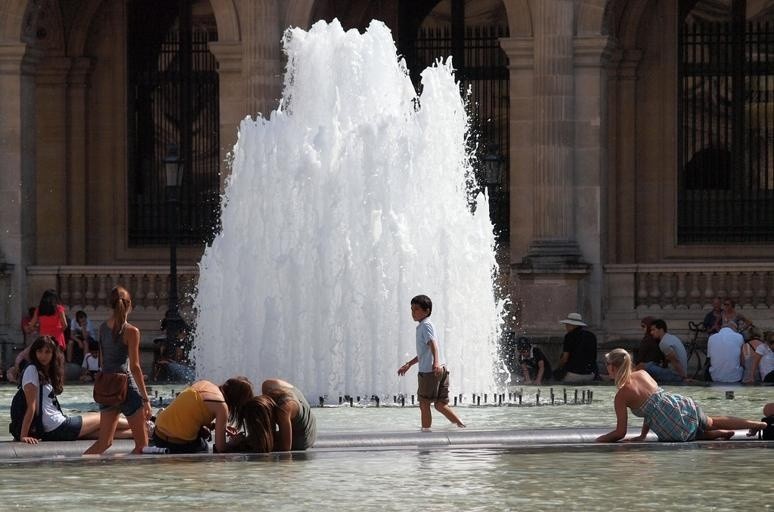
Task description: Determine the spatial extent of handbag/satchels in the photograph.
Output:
[93,372,128,405]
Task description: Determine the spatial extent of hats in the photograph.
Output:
[559,313,587,327]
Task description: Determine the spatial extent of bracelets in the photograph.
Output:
[142,397,154,402]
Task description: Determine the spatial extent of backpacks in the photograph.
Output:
[9,363,45,441]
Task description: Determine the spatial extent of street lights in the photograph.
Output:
[152,152,190,355]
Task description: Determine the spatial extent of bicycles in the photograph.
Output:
[680,321,755,381]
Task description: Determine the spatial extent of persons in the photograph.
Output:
[551,313,604,384]
[397,295,468,430]
[18,336,165,444]
[632,297,773,386]
[759,403,774,439]
[83,287,148,453]
[152,376,316,453]
[150,309,196,381]
[6,287,103,384]
[518,337,551,385]
[595,348,767,442]
